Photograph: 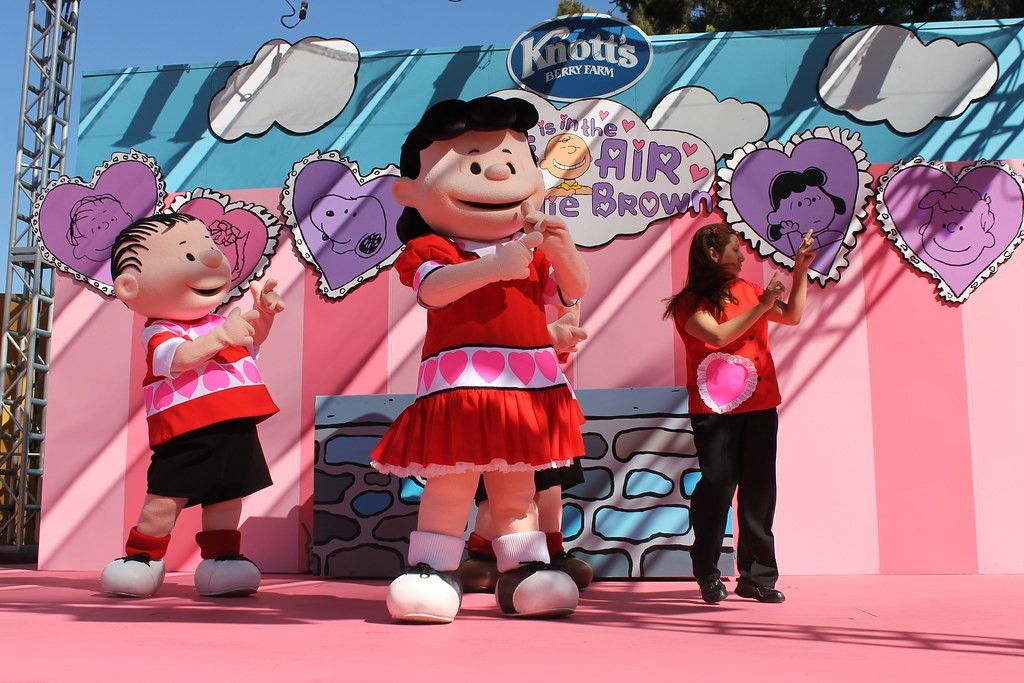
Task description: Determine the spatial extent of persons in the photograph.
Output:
[387,101,592,623]
[662,223,817,603]
[101,214,285,598]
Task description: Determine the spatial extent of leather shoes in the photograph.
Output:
[700,579,728,603]
[734,574,786,603]
